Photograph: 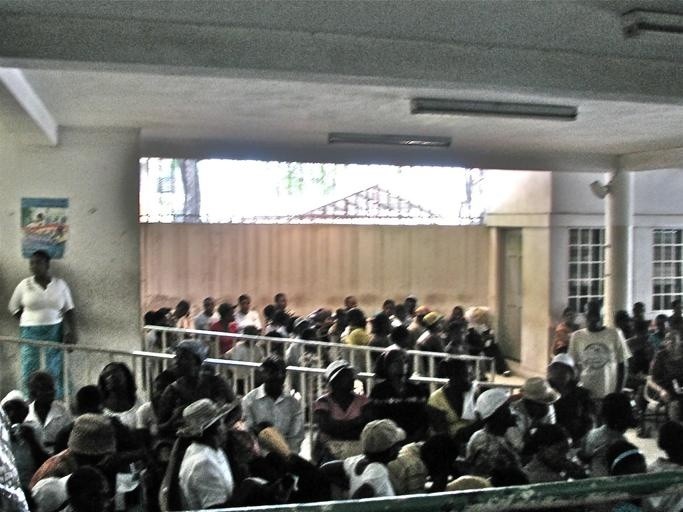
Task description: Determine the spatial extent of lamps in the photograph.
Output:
[326,133,451,147]
[408,96,579,123]
[619,6,682,42]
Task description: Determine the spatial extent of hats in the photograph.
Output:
[255,353,286,371]
[141,293,493,347]
[361,418,407,455]
[472,387,524,422]
[513,376,562,411]
[175,397,238,439]
[321,358,355,387]
[67,414,119,456]
[547,352,577,381]
[30,472,72,512]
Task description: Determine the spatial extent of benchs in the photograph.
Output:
[155,433,190,512]
